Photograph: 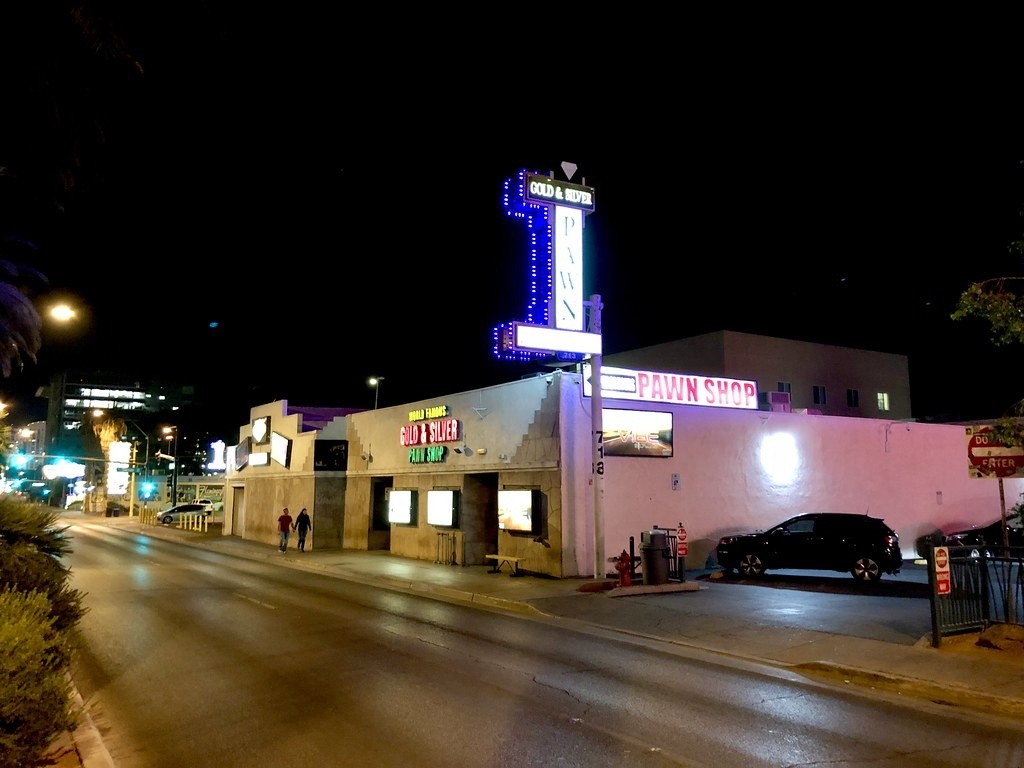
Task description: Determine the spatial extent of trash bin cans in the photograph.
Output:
[638,530,671,585]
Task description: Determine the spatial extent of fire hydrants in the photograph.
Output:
[615,549,631,586]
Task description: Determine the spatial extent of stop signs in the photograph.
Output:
[677,529,688,541]
[967,426,1024,478]
[935,549,948,568]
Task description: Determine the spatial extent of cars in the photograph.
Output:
[716,505,904,582]
[190,499,213,515]
[945,513,1024,558]
[156,505,206,524]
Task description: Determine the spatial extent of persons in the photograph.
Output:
[294,508,311,552]
[277,508,294,554]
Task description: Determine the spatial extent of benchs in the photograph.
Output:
[485,554,527,578]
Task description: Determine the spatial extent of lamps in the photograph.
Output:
[453,445,467,454]
[359,451,371,461]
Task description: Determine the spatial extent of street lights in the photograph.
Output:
[368,376,385,409]
[33,301,76,321]
[163,426,177,506]
